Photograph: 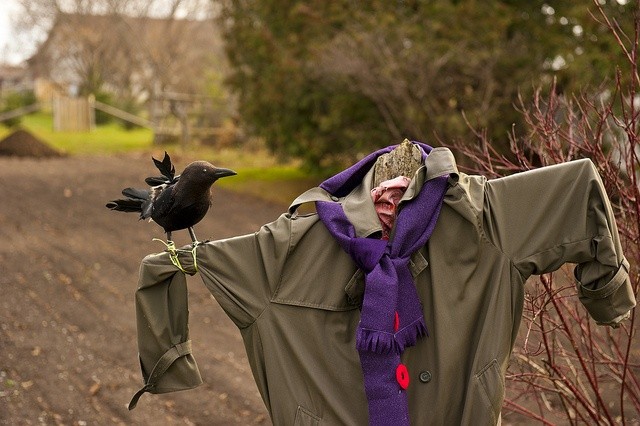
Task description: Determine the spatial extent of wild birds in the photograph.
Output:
[106,151,236,252]
